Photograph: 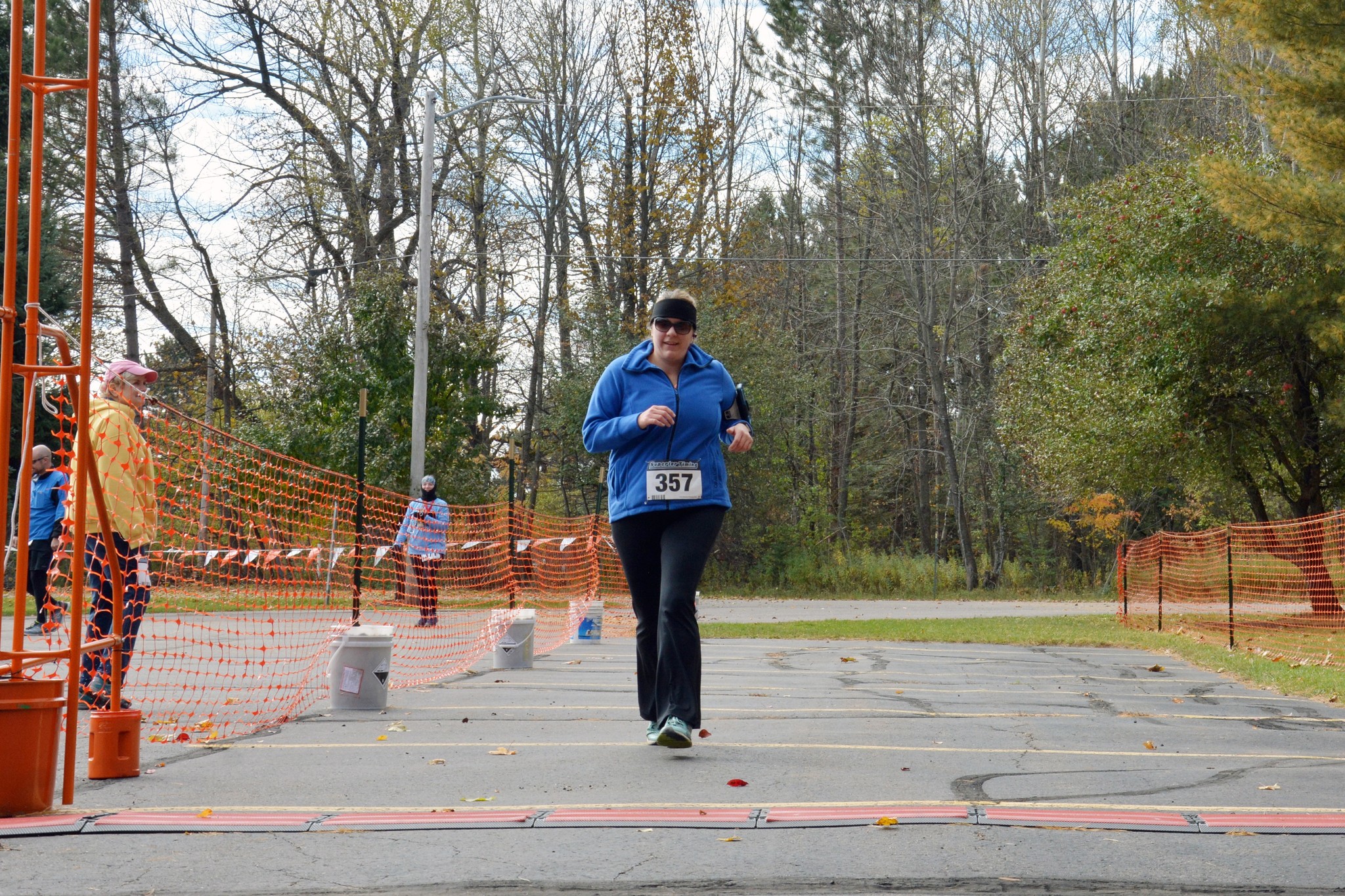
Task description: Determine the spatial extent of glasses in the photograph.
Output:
[32,455,49,465]
[653,317,692,335]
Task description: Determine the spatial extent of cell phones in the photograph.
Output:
[738,383,749,415]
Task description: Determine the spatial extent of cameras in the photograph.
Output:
[692,333,697,338]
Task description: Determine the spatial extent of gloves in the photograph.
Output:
[413,512,426,522]
[391,545,400,558]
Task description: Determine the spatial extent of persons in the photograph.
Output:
[25,444,71,634]
[68,360,160,708]
[580,290,753,747]
[394,475,452,627]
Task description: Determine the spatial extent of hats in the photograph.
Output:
[105,359,158,386]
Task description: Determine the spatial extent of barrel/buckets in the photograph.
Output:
[569,600,604,644]
[489,608,536,668]
[325,625,394,710]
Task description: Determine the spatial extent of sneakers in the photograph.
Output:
[77,676,132,710]
[24,620,52,636]
[50,600,70,632]
[414,616,426,627]
[424,616,439,627]
[646,715,692,748]
